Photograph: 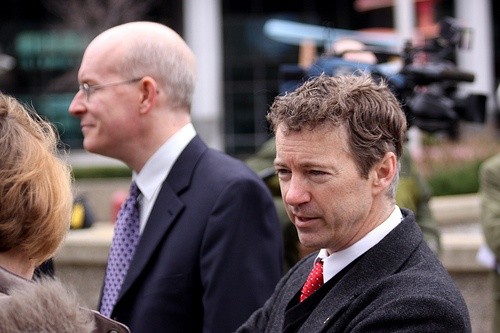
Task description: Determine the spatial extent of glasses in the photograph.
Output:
[77,76,160,100]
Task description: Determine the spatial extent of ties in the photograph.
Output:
[99,180,141,318]
[298,260,324,303]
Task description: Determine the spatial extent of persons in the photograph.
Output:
[295,14,458,88]
[67,20,287,333]
[1,90,130,333]
[232,69,471,333]
